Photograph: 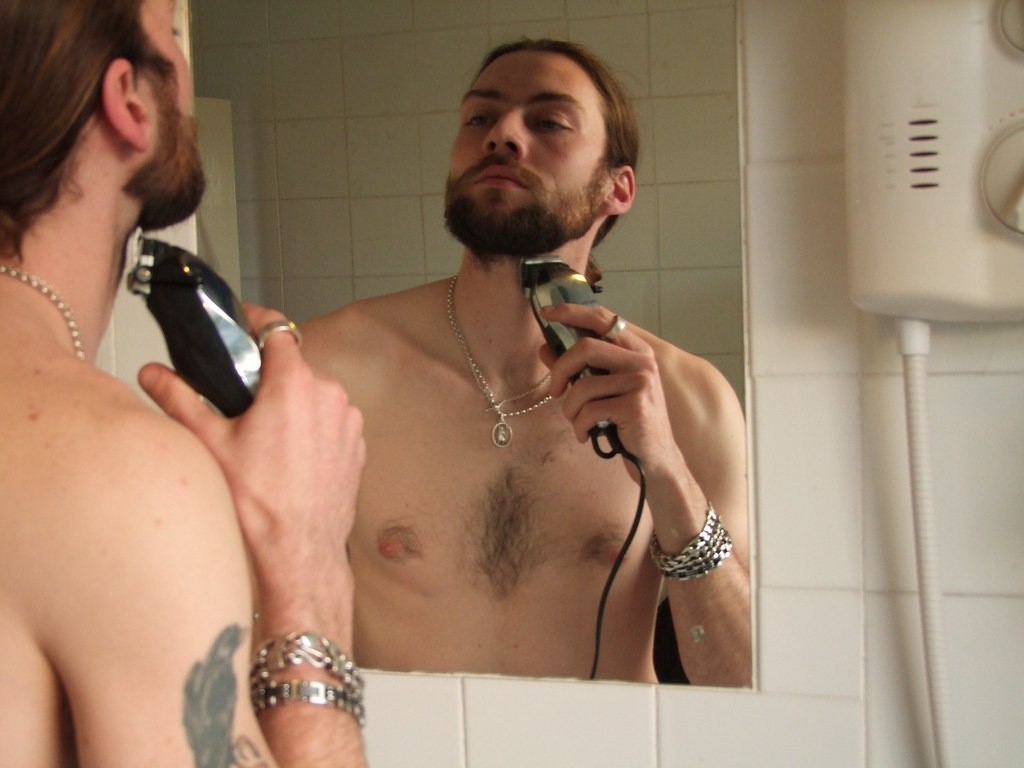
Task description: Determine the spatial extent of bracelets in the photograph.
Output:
[251,629,366,728]
[650,500,732,581]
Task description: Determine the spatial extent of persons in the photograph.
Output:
[294,36,753,690]
[0,0,369,768]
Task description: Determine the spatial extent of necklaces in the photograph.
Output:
[0,262,86,360]
[447,272,551,449]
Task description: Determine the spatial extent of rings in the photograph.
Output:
[258,319,302,352]
[598,314,626,342]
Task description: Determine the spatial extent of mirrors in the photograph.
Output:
[186,1,756,691]
